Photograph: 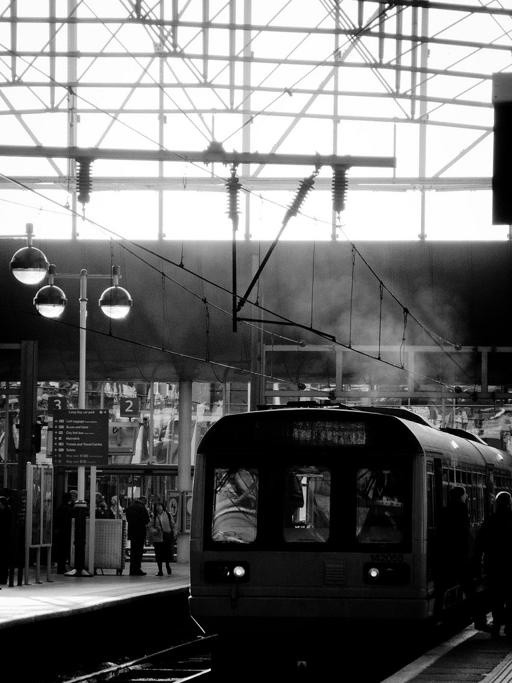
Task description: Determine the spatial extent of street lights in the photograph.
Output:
[0,221,49,286]
[33,261,136,580]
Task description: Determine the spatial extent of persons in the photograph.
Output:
[1,496,18,585]
[445,487,492,633]
[95,492,177,576]
[473,491,512,643]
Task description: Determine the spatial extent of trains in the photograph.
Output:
[187,398,512,672]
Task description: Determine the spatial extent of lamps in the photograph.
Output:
[10,224,48,286]
[99,263,132,318]
[34,264,66,318]
